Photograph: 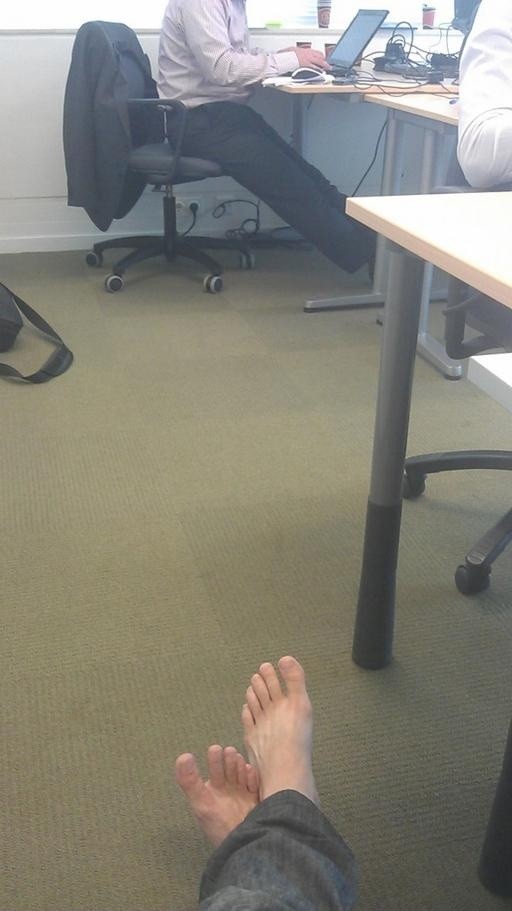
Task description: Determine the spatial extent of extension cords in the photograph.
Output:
[372,57,427,75]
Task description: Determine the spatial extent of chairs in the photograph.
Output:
[78,21,230,295]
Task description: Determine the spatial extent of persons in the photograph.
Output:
[174,655,362,911]
[156,0,377,285]
[455,0,511,191]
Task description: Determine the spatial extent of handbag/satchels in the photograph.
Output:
[0,282,73,383]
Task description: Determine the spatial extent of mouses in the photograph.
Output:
[291,67,320,79]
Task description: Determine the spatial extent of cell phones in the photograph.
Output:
[332,78,357,86]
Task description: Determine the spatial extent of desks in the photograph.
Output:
[259,65,462,380]
[341,190,512,672]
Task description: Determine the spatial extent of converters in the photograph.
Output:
[385,41,403,59]
[233,233,279,249]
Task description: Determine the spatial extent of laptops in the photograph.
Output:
[281,9,389,77]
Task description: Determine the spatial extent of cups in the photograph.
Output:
[296,41,312,49]
[422,7,436,29]
[317,0,331,29]
[325,43,335,57]
